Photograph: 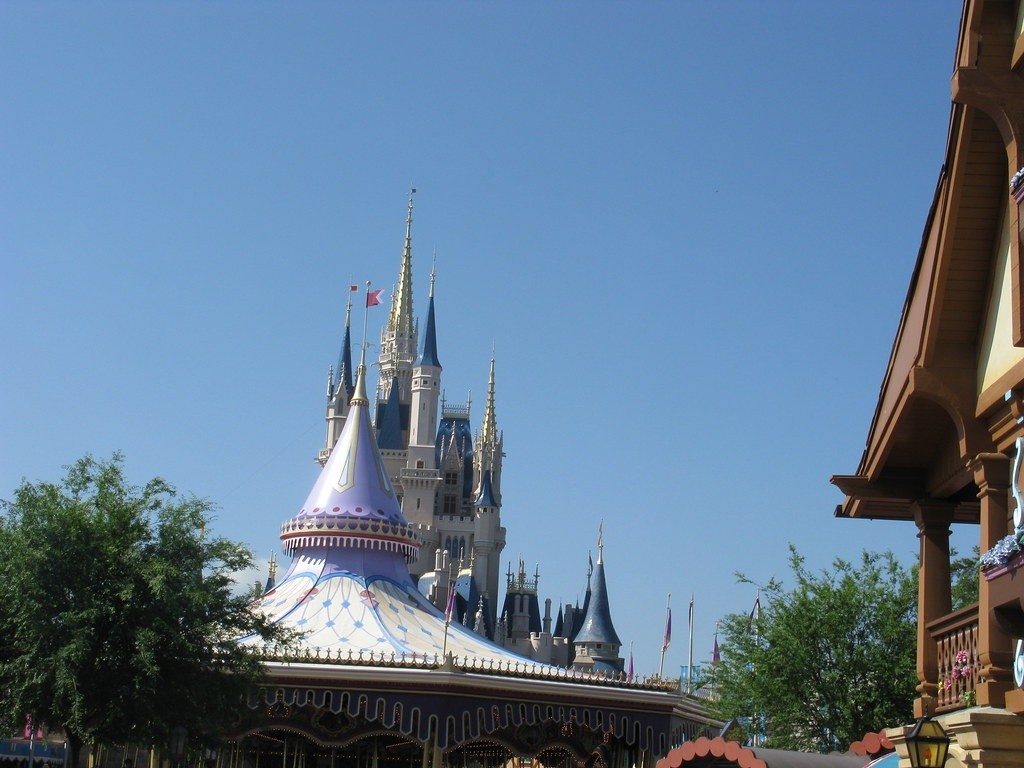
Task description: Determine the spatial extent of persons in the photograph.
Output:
[123,759,133,768]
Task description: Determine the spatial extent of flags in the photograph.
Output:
[688,601,694,628]
[366,289,385,306]
[350,285,358,292]
[445,578,456,625]
[663,606,672,652]
[411,189,416,194]
[713,636,720,662]
[630,649,635,678]
